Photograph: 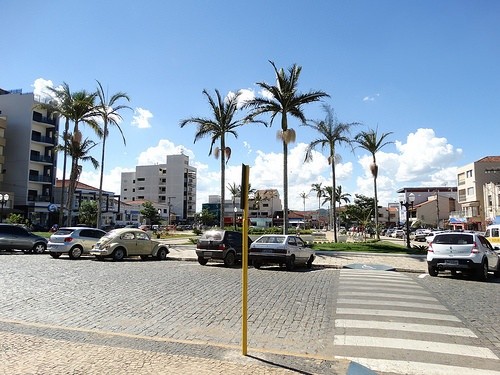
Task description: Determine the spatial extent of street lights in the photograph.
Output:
[158,209,161,228]
[233,206,238,230]
[398,193,415,248]
[0,193,10,223]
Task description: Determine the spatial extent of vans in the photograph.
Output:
[482,224,500,250]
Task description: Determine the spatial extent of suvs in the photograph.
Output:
[425,231,500,281]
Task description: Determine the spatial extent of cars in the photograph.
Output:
[126,224,202,230]
[347,226,406,239]
[247,234,317,271]
[195,230,255,269]
[0,224,48,254]
[88,228,170,262]
[45,227,108,262]
[409,227,486,242]
